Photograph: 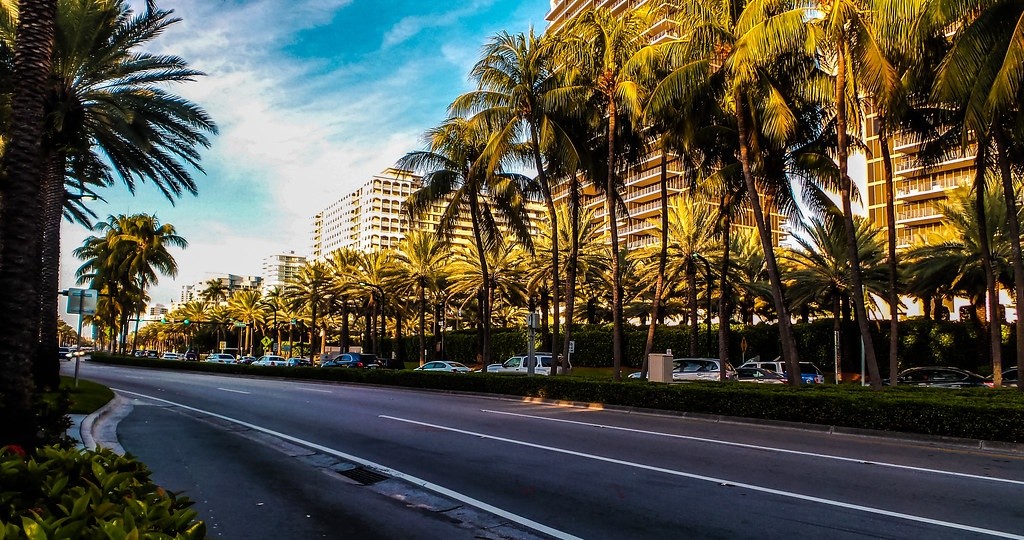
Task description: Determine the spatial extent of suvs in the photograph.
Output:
[378,358,406,370]
[475,355,563,375]
[740,361,825,385]
[321,352,384,369]
[181,352,199,361]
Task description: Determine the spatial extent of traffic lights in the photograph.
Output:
[184,320,189,324]
[161,319,166,323]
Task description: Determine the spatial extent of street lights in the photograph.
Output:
[358,282,385,358]
[692,253,712,357]
[260,301,276,355]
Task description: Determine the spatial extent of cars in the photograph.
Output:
[251,355,290,366]
[236,356,256,365]
[125,350,159,358]
[161,353,178,360]
[70,345,99,357]
[863,367,995,389]
[736,368,789,384]
[414,360,473,372]
[986,368,1019,389]
[204,353,238,364]
[628,357,739,383]
[59,347,70,361]
[287,358,313,367]
